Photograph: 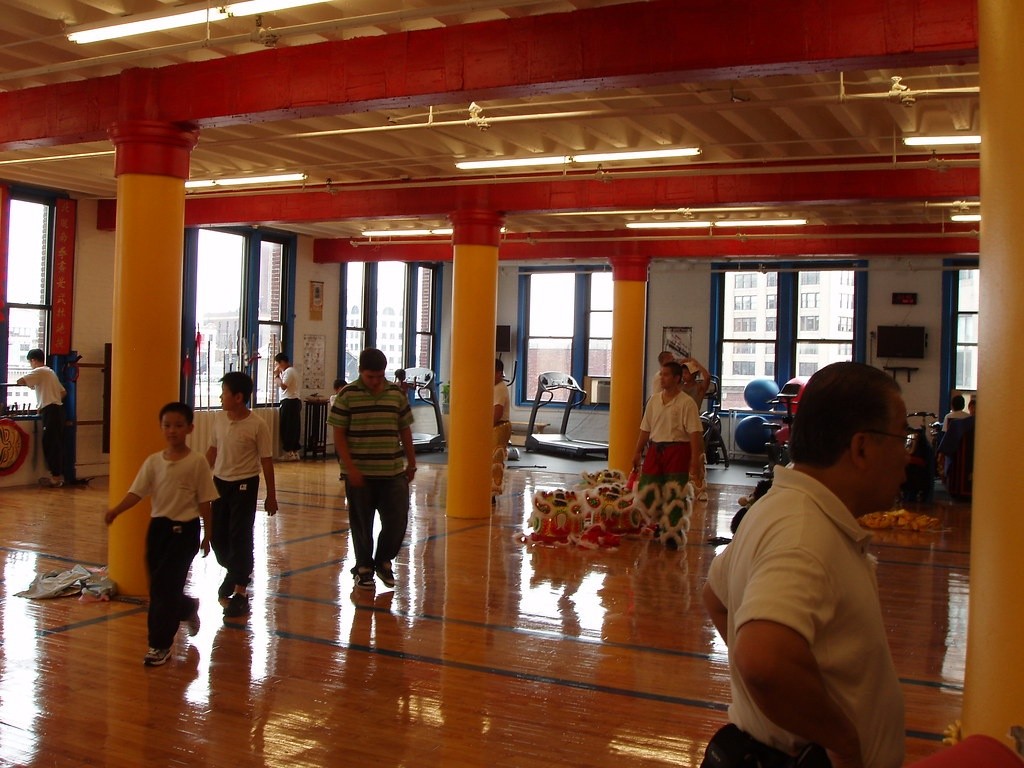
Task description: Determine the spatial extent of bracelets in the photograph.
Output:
[275,375,279,378]
[406,466,417,472]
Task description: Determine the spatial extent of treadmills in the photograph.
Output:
[393,368,447,452]
[525,372,609,460]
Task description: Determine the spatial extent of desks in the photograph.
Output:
[303,397,330,458]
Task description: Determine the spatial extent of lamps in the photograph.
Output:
[903,134,983,147]
[361,225,509,237]
[184,172,308,189]
[948,213,981,222]
[453,144,702,171]
[624,219,713,230]
[67,0,331,46]
[714,218,811,228]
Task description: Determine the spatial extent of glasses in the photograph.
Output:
[863,428,920,455]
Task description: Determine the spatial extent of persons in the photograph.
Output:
[491,358,511,505]
[699,362,912,768]
[652,351,676,395]
[18,349,66,486]
[968,400,976,417]
[330,379,347,479]
[633,362,703,548]
[105,402,219,665]
[327,348,416,589]
[394,369,416,397]
[941,396,968,433]
[206,372,278,615]
[680,358,711,501]
[275,353,302,461]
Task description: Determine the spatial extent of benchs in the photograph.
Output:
[510,421,551,446]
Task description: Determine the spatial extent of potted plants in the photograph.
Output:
[440,382,451,414]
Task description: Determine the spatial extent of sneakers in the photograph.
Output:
[376,562,395,586]
[354,571,376,590]
[278,452,300,461]
[187,597,201,637]
[223,593,250,615]
[218,572,236,597]
[143,648,172,667]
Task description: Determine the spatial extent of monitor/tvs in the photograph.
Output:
[496,325,510,352]
[876,326,924,359]
